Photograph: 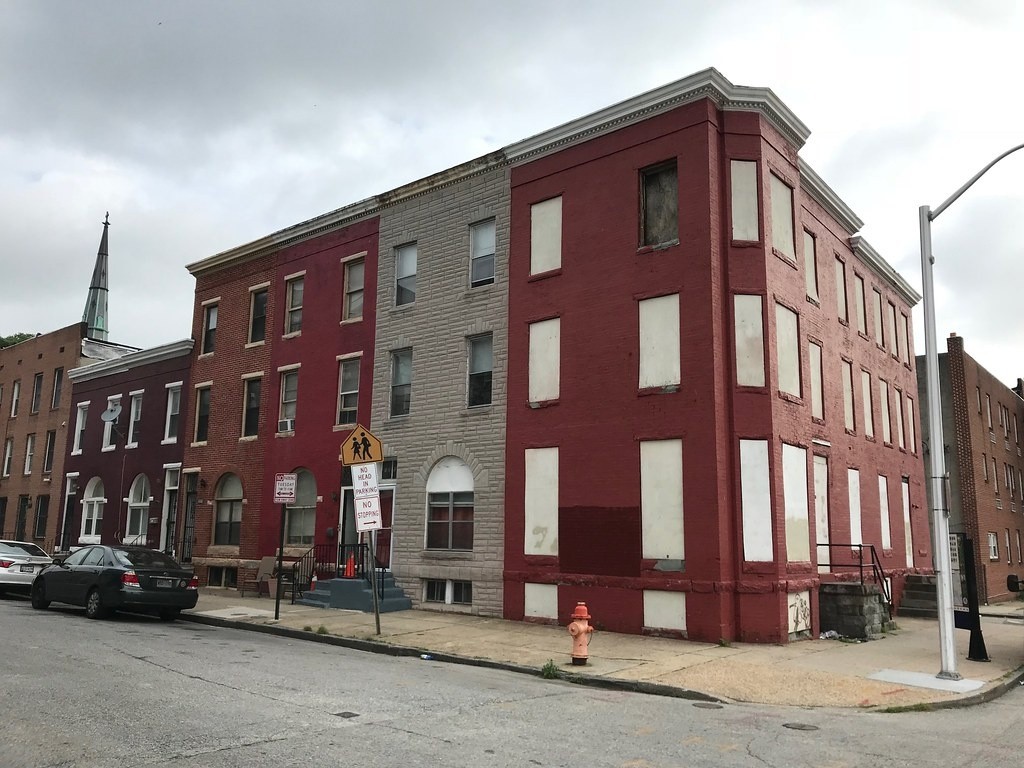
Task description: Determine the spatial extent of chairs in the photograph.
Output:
[274,558,316,599]
[241,557,275,597]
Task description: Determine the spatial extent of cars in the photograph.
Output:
[29,545,199,622]
[0,540,55,596]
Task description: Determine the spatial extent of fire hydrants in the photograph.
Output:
[567,601,596,666]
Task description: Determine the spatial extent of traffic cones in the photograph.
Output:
[342,552,358,579]
[310,571,319,590]
